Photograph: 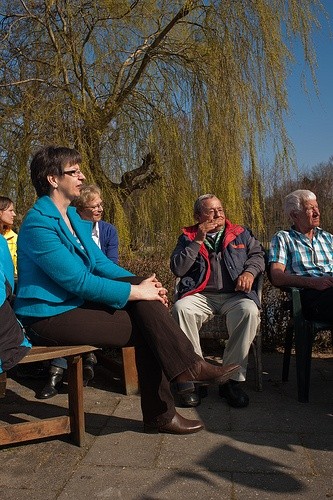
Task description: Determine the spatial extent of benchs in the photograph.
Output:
[0,345,138,455]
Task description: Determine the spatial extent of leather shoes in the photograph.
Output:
[172,360,240,392]
[219,379,249,407]
[82,352,96,387]
[178,382,201,407]
[144,412,204,435]
[34,372,64,399]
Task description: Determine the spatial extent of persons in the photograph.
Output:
[269,190,333,325]
[14,146,242,435]
[170,194,268,407]
[0,185,120,399]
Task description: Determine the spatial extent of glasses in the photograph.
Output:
[82,202,105,211]
[64,168,83,177]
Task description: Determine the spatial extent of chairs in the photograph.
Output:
[281,286,333,403]
[175,270,265,392]
